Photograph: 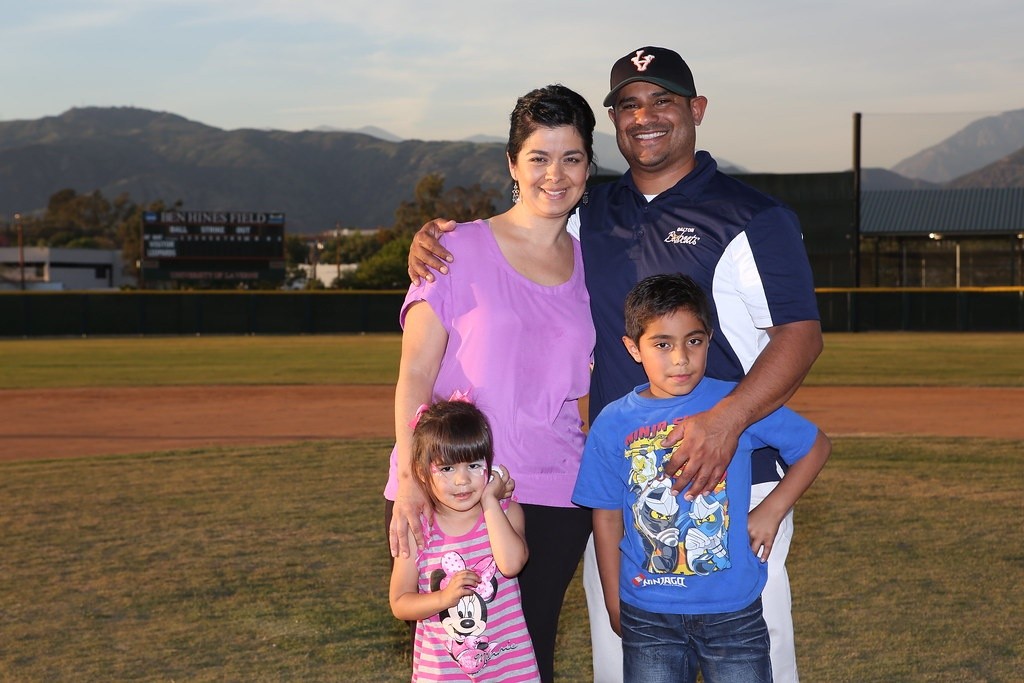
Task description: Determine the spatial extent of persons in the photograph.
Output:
[571,274,830,683]
[382,85,596,683]
[387,391,549,683]
[406,46,824,683]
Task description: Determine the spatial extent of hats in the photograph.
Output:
[603,46,697,107]
